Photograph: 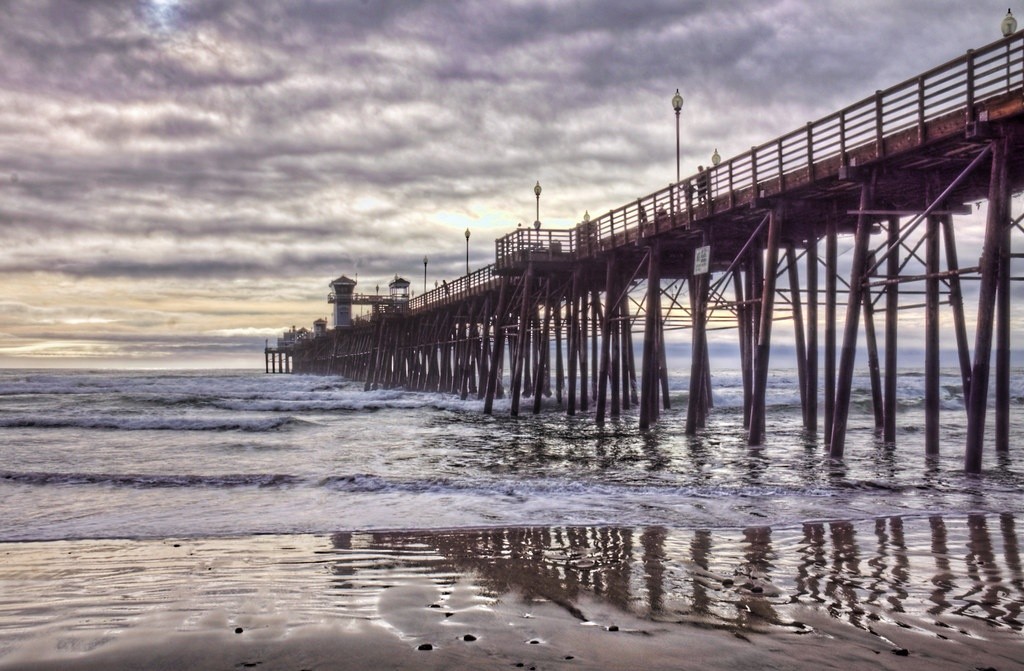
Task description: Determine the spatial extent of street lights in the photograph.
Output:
[671,90,684,211]
[423,256,428,305]
[712,147,721,197]
[1001,7,1018,91]
[465,228,471,288]
[534,182,541,248]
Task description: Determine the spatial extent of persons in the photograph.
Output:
[680,181,697,212]
[441,280,450,298]
[697,166,707,206]
[657,202,668,218]
[640,207,647,223]
